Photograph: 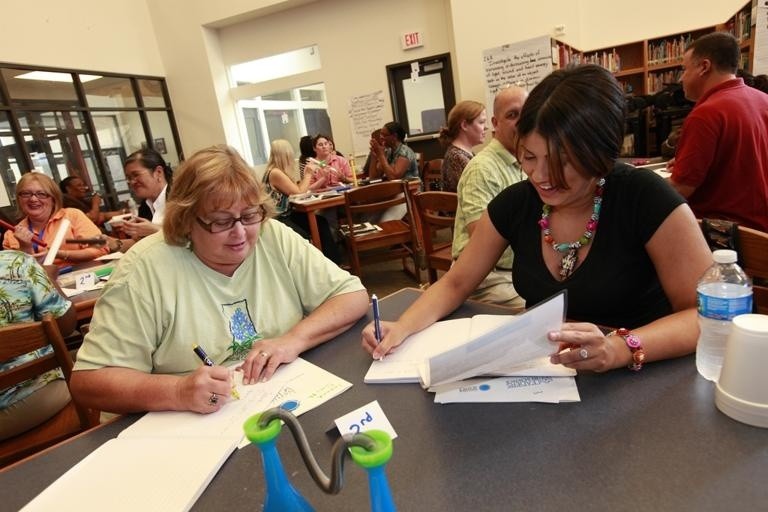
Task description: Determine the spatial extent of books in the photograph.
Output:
[556,12,752,154]
[365,314,580,383]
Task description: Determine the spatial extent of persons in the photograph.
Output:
[360,67,721,375]
[1,81,768,313]
[663,32,768,314]
[0,250,78,441]
[69,144,369,414]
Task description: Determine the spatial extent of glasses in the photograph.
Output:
[19,190,52,199]
[194,210,266,233]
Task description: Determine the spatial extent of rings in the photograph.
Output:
[259,352,268,357]
[579,348,588,359]
[208,391,218,406]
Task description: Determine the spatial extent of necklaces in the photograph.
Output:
[539,178,605,281]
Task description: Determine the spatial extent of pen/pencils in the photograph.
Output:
[371,293,383,361]
[192,343,240,400]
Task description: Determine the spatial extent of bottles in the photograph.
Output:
[694,249,756,382]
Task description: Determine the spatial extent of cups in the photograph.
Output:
[714,312,768,430]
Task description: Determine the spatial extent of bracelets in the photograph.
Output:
[604,327,646,370]
